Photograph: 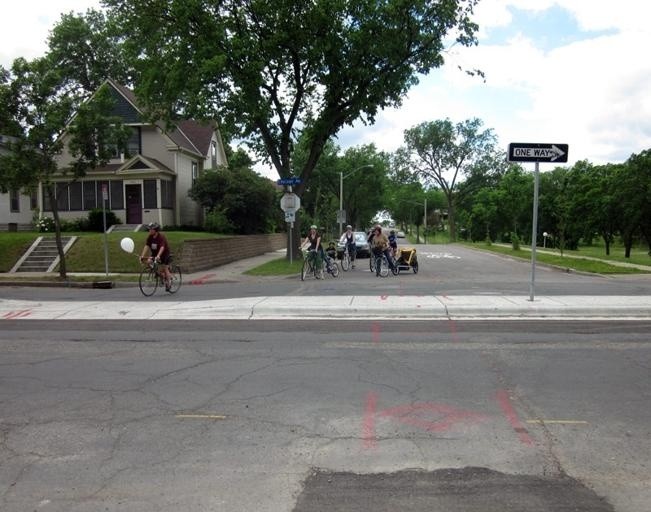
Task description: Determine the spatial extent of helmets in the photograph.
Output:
[311,225,317,229]
[147,222,159,229]
[346,225,352,229]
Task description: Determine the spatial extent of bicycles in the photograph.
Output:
[139,256,182,296]
[301,248,339,281]
[369,245,394,277]
[341,242,357,271]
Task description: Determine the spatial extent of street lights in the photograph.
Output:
[543,232,548,248]
[339,164,374,235]
[392,197,427,230]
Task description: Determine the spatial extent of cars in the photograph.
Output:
[337,232,372,259]
[397,231,405,238]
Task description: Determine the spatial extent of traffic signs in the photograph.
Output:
[508,142,569,162]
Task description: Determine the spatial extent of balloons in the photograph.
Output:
[120,236,135,254]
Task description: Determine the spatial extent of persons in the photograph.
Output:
[140,222,173,291]
[325,225,357,268]
[298,225,323,279]
[367,225,397,277]
